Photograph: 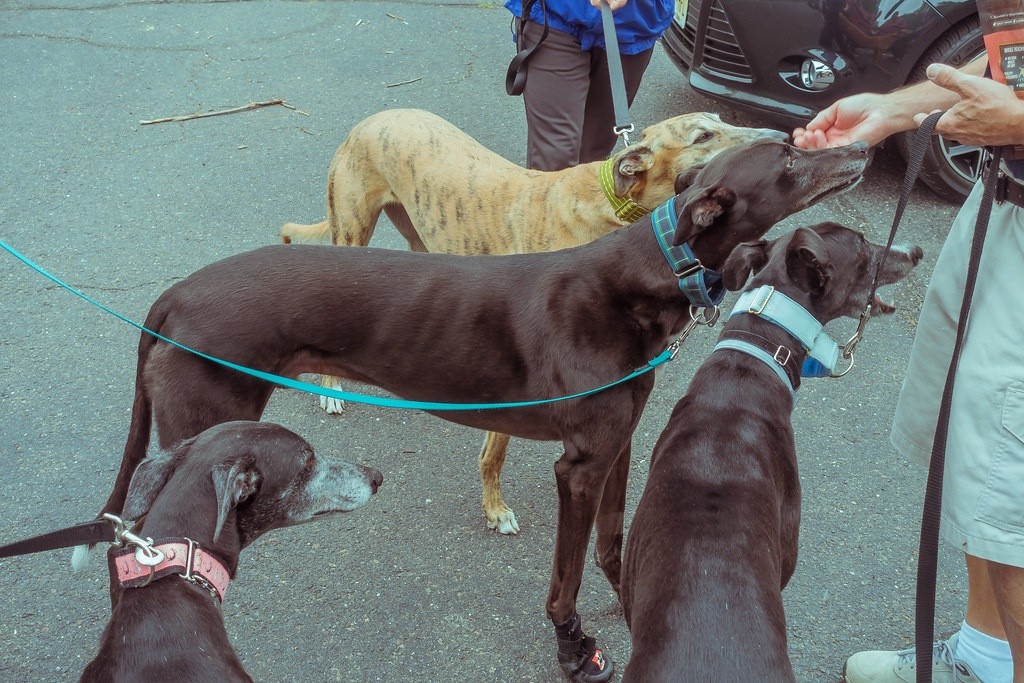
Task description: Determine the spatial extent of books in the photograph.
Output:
[975,0,1024,160]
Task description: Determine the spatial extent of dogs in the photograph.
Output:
[79,420,384,683]
[619,222,926,683]
[280,107,791,539]
[1,139,870,683]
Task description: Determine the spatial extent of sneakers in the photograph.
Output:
[841,631,984,683]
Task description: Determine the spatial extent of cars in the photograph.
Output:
[656,0,993,208]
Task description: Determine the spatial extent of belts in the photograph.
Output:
[981,151,1024,208]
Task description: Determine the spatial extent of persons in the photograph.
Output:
[505,0,675,172]
[794,52,1024,683]
